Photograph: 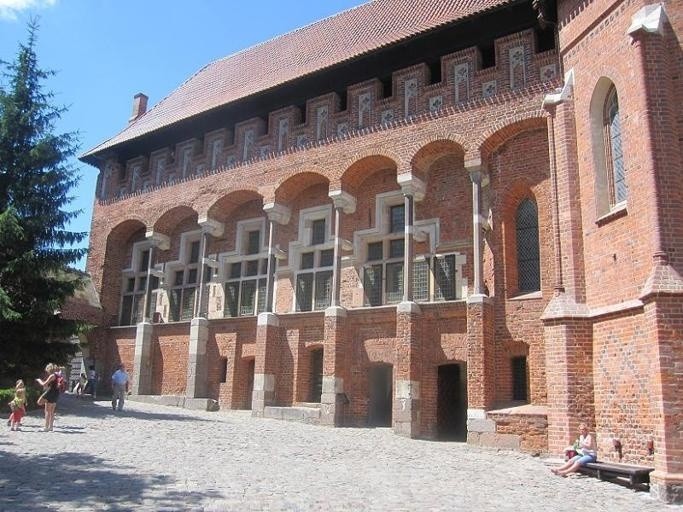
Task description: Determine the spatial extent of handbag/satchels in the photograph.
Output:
[37,393,47,405]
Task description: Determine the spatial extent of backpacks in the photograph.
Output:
[10,400,18,412]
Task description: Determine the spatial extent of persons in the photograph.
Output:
[111,363,129,412]
[6,378,28,431]
[82,364,96,399]
[550,423,596,476]
[55,366,65,392]
[75,372,87,398]
[35,362,58,432]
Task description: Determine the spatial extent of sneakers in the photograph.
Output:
[38,428,48,432]
[7,421,22,431]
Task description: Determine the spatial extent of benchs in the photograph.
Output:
[585,461,655,491]
